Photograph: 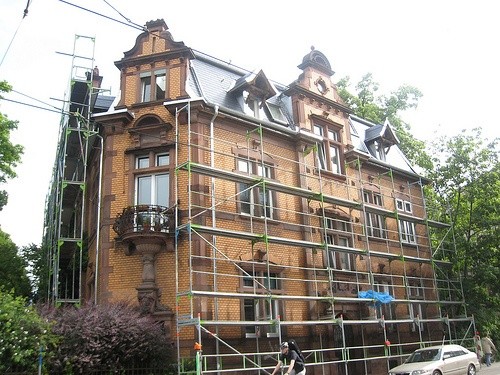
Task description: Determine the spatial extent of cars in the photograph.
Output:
[388,344,481,375]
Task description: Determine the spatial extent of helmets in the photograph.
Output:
[281,342,289,349]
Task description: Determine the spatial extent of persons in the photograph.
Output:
[270,341,306,375]
[481,333,496,366]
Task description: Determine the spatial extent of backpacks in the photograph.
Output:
[289,338,306,362]
